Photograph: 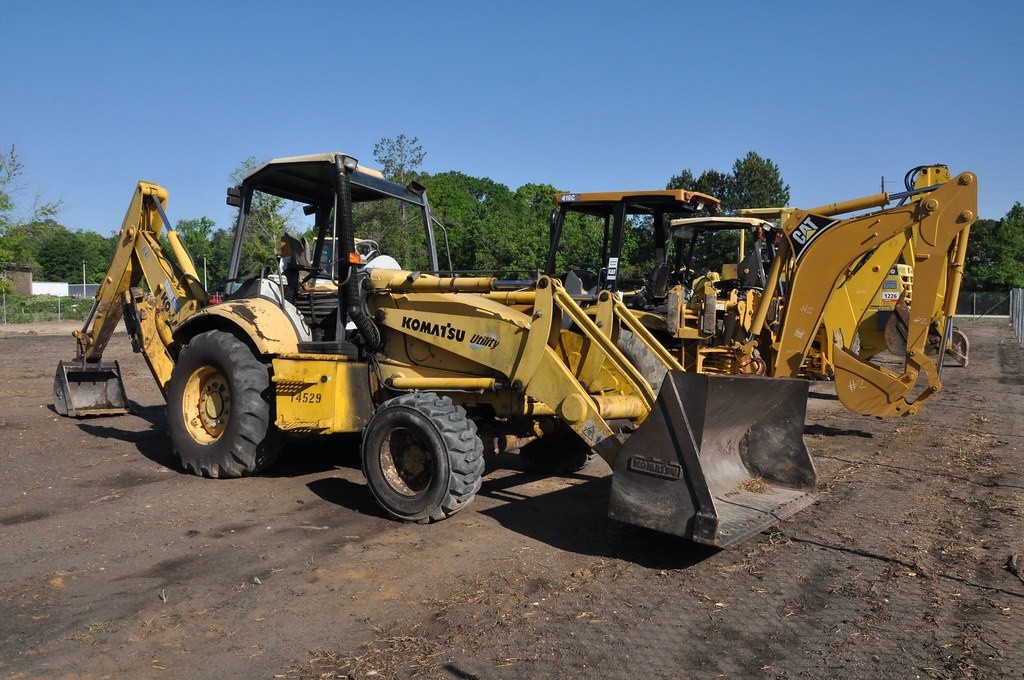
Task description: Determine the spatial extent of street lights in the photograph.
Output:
[200,252,210,294]
[82,259,88,303]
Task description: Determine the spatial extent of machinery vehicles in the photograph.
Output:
[50,151,824,551]
[469,163,979,424]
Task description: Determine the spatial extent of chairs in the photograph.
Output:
[624,263,671,304]
[282,231,335,299]
[714,250,759,299]
